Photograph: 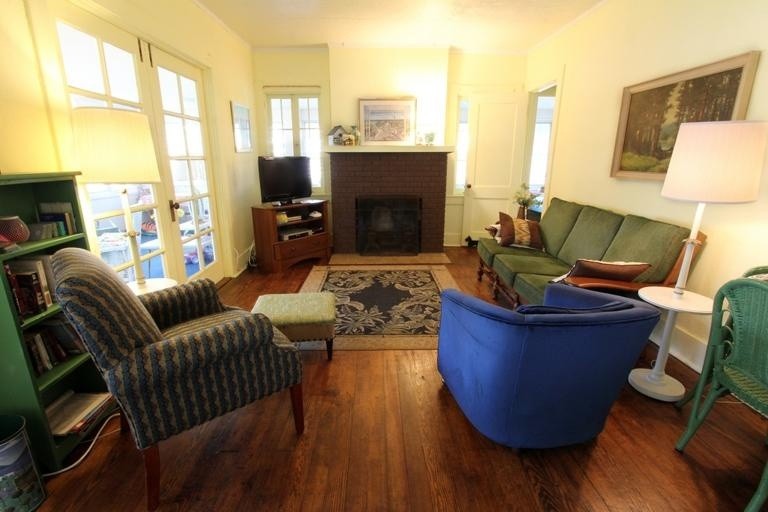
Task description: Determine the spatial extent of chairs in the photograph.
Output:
[670,265,767,408]
[435,282,662,450]
[675,277,767,512]
[48,245,305,512]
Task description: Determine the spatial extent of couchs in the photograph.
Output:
[475,197,706,311]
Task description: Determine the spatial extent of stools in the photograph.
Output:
[249,291,337,362]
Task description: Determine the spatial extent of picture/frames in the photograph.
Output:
[357,96,417,147]
[229,100,254,154]
[608,48,763,182]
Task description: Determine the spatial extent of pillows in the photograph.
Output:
[498,211,545,253]
[547,257,652,285]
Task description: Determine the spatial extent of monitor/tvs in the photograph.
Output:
[258,156,312,205]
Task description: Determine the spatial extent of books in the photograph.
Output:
[3,201,114,440]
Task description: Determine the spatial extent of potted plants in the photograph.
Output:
[511,181,546,219]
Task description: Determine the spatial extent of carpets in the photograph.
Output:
[295,264,463,353]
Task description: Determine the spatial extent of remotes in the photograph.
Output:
[272,202,279,206]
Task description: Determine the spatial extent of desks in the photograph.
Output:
[125,278,178,297]
[626,286,713,403]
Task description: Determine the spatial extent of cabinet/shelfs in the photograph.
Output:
[249,197,330,274]
[0,168,119,481]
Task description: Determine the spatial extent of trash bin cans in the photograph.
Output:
[0,414,47,511]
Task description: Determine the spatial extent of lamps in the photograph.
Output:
[68,104,162,285]
[656,119,767,297]
